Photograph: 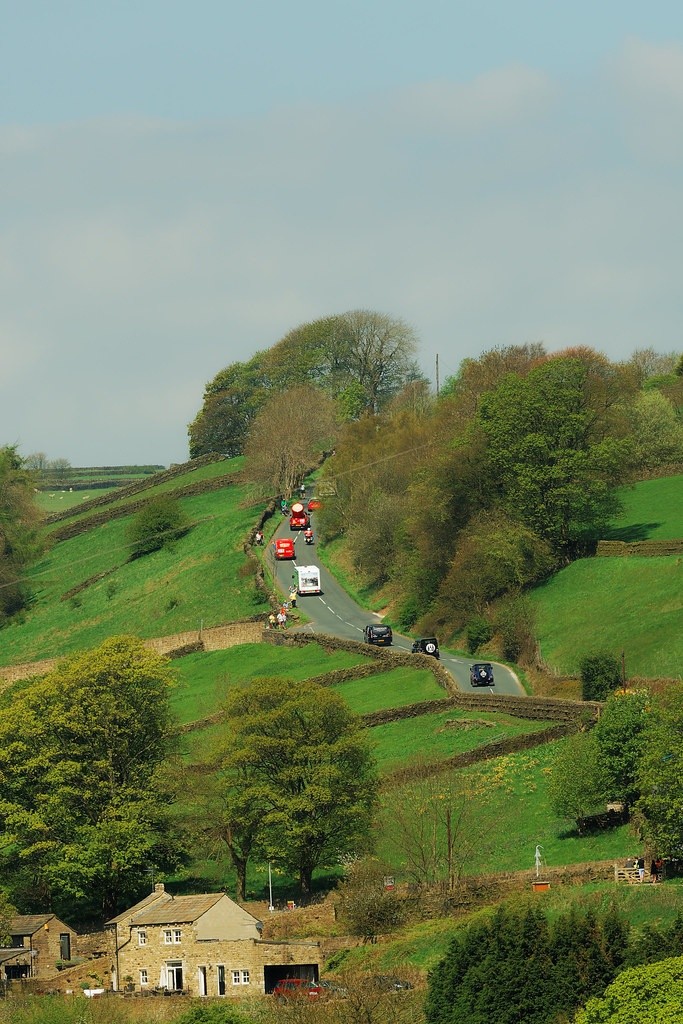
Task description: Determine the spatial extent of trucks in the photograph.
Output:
[291,565,322,596]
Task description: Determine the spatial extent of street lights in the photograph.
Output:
[535,844,544,877]
[268,860,274,915]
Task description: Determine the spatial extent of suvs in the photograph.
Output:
[362,624,393,647]
[469,663,495,688]
[411,637,440,659]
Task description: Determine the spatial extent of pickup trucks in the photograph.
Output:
[288,511,311,530]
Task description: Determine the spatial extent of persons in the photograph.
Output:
[623,855,682,884]
[299,483,305,499]
[254,499,314,546]
[269,589,298,630]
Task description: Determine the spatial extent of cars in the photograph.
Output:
[272,977,329,1006]
[360,974,410,993]
[315,978,348,1000]
[306,499,322,512]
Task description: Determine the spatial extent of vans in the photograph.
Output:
[273,538,296,561]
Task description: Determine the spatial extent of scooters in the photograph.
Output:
[303,531,313,545]
